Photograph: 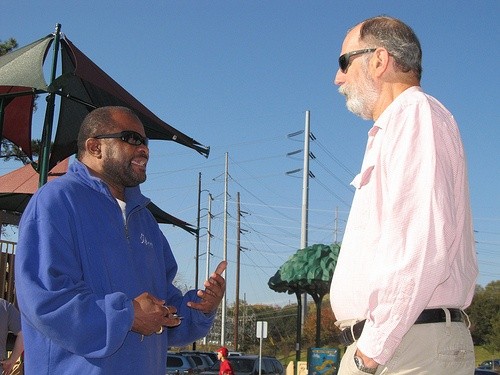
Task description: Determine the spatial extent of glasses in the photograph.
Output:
[95,130,149,147]
[338,49,376,73]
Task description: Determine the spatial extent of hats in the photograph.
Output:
[215,347,228,357]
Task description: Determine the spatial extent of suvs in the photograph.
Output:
[199,355,283,375]
[165,351,245,375]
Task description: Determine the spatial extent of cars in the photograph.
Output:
[474,359,500,375]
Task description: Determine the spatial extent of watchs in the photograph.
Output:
[354,356,377,374]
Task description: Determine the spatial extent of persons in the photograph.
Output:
[217,347,234,375]
[15,106,228,375]
[0,297,24,375]
[329,15,479,375]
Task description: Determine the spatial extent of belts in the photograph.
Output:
[339,308,464,348]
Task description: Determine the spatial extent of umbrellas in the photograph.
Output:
[0,151,201,235]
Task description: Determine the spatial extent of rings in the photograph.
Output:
[163,305,171,314]
[155,325,164,335]
